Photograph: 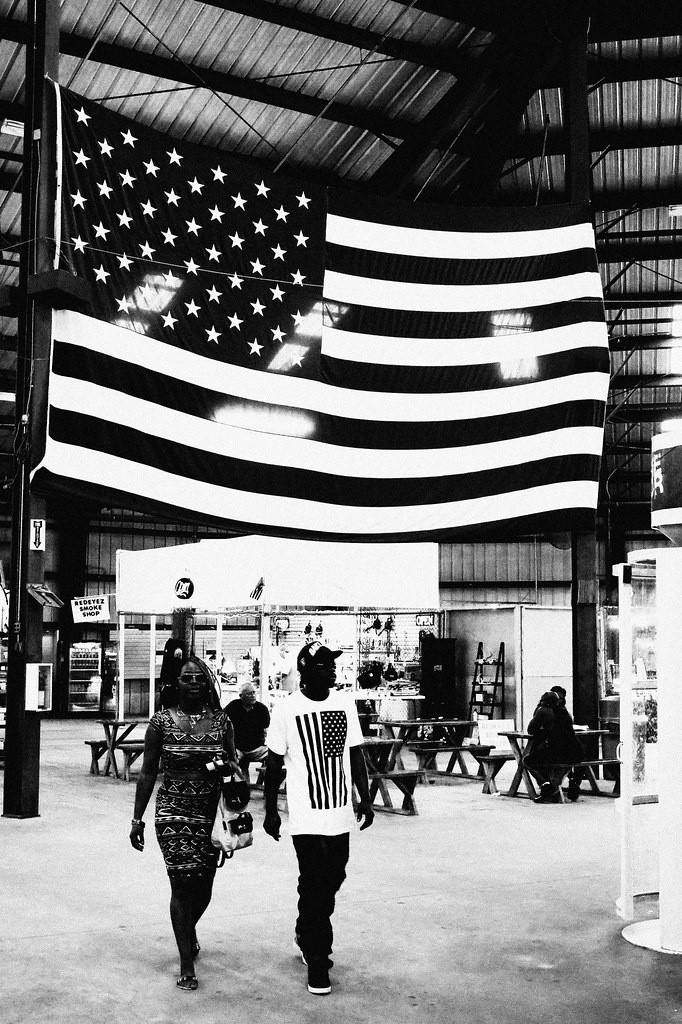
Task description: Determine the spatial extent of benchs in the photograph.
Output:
[538,758,622,803]
[363,769,424,817]
[86,739,147,782]
[407,738,531,798]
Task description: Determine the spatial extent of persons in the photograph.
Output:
[522,686,588,803]
[264,642,374,994]
[222,682,270,775]
[130,656,241,991]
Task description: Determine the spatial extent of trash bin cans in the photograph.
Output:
[599,696,621,780]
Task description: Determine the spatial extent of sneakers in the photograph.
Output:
[308,962,331,993]
[302,951,333,969]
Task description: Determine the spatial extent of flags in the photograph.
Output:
[45,81,611,542]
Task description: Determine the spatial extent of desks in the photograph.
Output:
[376,720,479,777]
[354,735,403,809]
[97,719,151,779]
[497,730,610,800]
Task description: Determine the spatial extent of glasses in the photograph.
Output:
[178,674,209,683]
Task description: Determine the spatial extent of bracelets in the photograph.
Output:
[132,820,145,828]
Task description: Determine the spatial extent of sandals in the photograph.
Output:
[191,929,200,955]
[177,964,198,990]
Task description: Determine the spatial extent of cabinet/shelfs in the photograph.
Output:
[468,642,505,720]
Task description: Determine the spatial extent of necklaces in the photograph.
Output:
[175,705,207,729]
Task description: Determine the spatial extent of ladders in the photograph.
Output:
[468,642,506,721]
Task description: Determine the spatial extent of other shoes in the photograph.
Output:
[534,783,560,803]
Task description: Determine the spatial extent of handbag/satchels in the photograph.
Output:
[210,761,253,852]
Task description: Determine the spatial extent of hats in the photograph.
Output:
[297,641,343,673]
